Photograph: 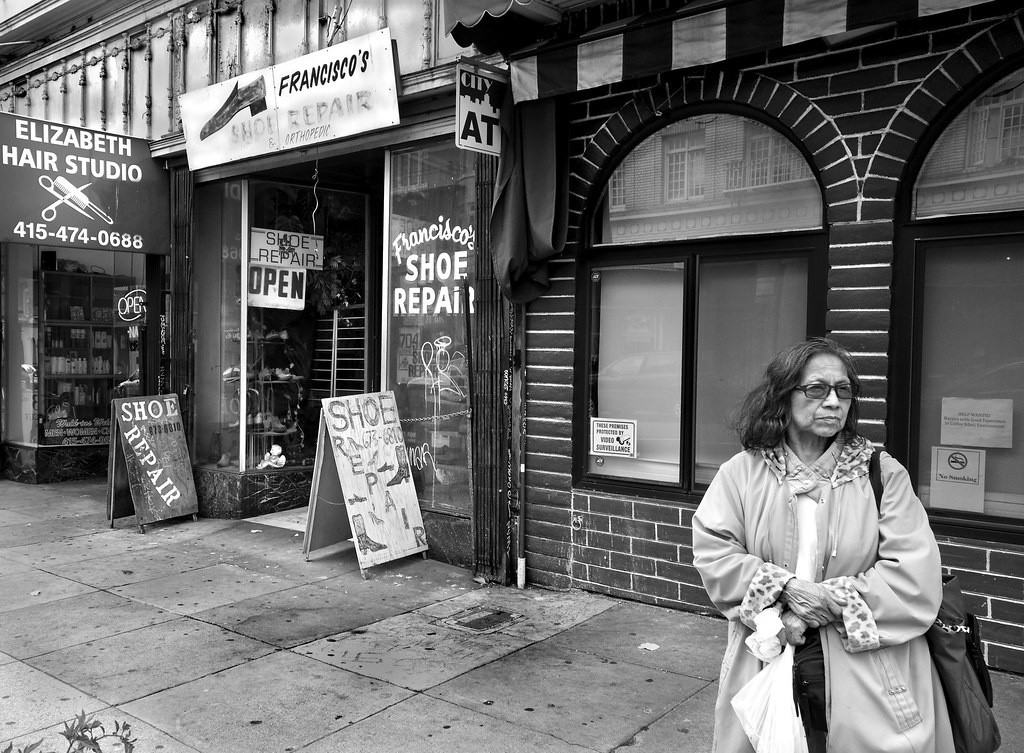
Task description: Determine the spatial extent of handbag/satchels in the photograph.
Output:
[868,449,1001,753]
[729,642,809,753]
[791,634,827,734]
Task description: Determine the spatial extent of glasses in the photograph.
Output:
[795,383,861,401]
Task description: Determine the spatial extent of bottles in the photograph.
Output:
[52,351,111,376]
[58,380,103,404]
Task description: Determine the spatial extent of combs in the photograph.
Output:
[54,176,114,223]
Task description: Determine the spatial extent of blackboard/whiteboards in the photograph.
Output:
[114,393,198,526]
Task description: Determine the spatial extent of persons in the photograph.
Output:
[691,337,957,753]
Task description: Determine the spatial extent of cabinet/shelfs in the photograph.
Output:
[222,340,301,469]
[37,269,131,420]
